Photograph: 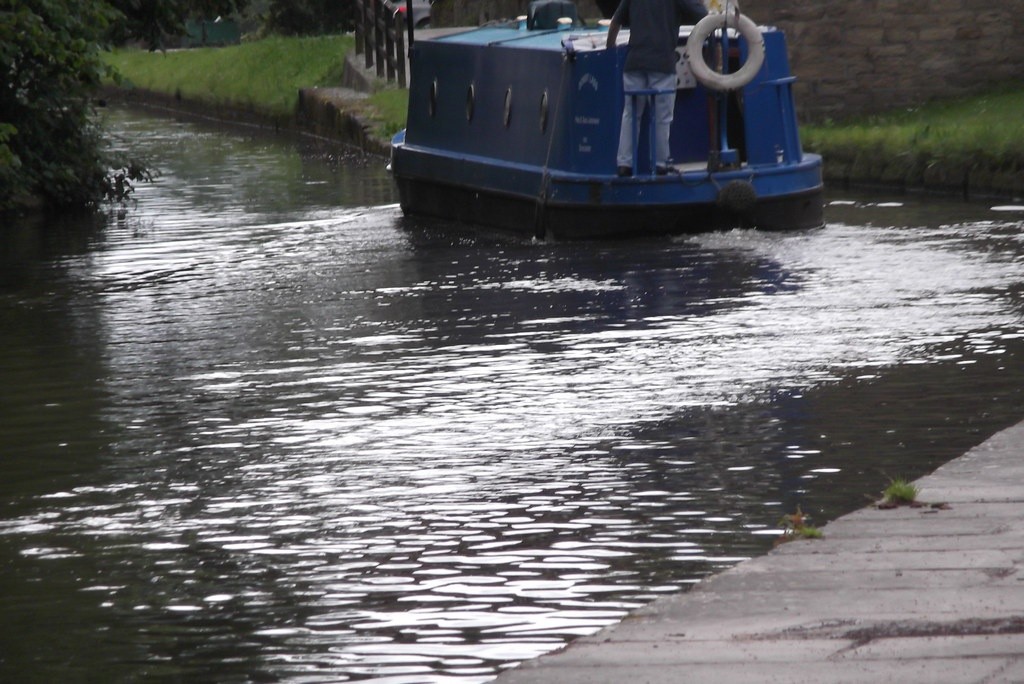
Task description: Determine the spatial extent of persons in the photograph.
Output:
[607,0,710,177]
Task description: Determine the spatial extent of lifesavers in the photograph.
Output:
[687,11,766,91]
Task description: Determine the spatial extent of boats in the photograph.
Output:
[386,16,824,238]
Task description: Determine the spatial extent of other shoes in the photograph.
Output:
[618,166,633,176]
[654,162,679,174]
